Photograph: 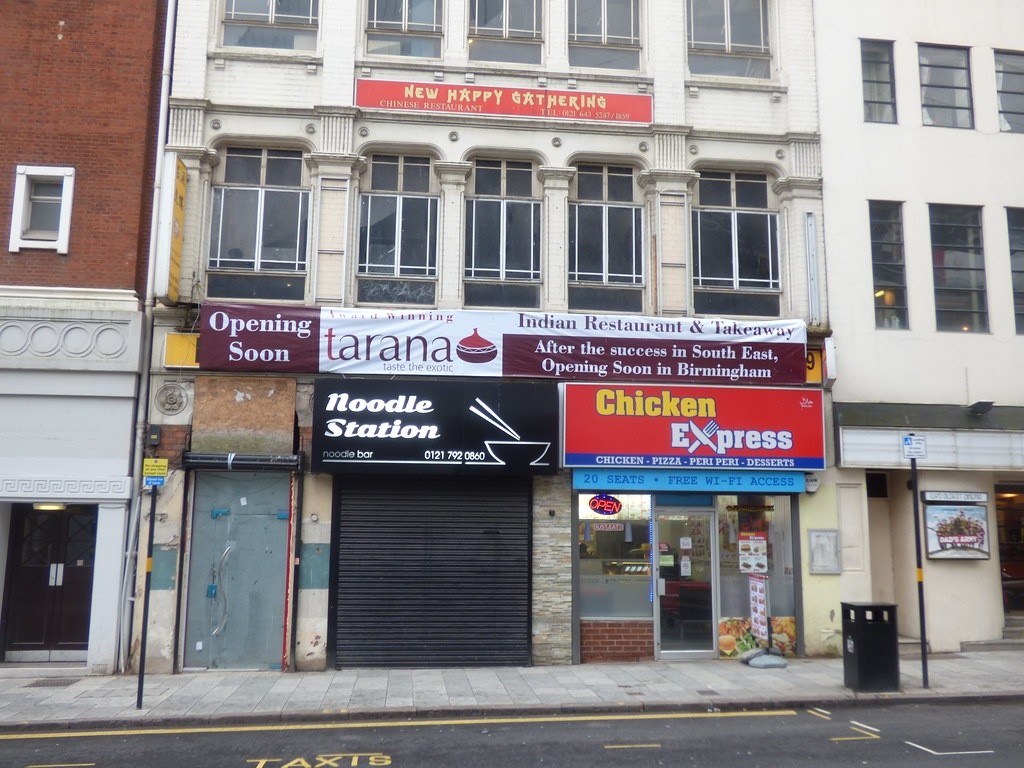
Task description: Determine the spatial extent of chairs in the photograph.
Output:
[660,582,709,634]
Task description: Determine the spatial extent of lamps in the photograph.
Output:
[969,400,995,415]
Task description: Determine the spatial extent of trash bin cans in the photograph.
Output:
[840,600,901,693]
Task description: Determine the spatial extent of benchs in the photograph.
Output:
[672,584,711,639]
